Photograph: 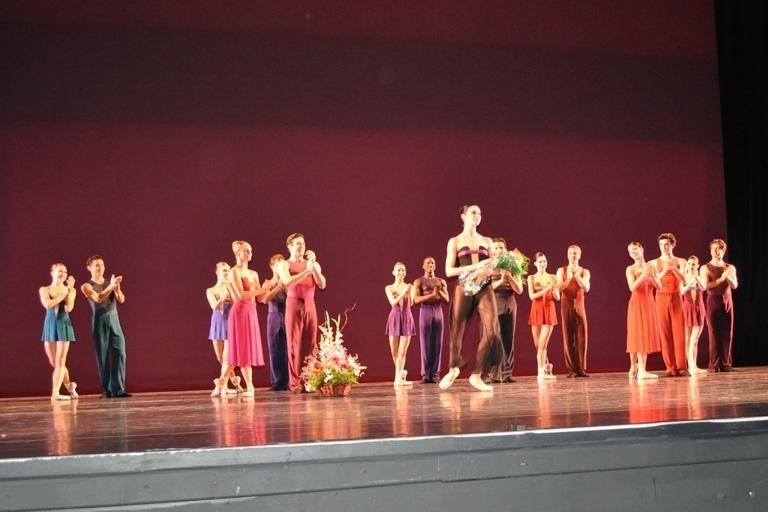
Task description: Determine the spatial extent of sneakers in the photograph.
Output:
[51,392,68,400]
[438,366,460,390]
[566,372,578,378]
[99,393,109,398]
[627,365,736,379]
[545,363,553,375]
[110,391,133,398]
[210,376,316,398]
[503,377,517,383]
[537,373,556,379]
[67,382,79,399]
[393,370,439,386]
[577,370,591,377]
[469,374,493,391]
[485,379,501,383]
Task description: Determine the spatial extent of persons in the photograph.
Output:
[622,241,664,380]
[483,238,524,383]
[556,244,592,380]
[435,204,502,394]
[39,262,81,401]
[260,253,290,392]
[411,257,450,384]
[80,253,133,398]
[385,261,419,382]
[212,238,283,395]
[204,261,243,398]
[526,250,564,383]
[698,239,740,373]
[277,233,327,393]
[647,234,692,377]
[679,255,710,373]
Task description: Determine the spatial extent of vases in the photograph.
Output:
[320,384,351,396]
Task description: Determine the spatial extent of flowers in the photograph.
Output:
[298,304,368,392]
